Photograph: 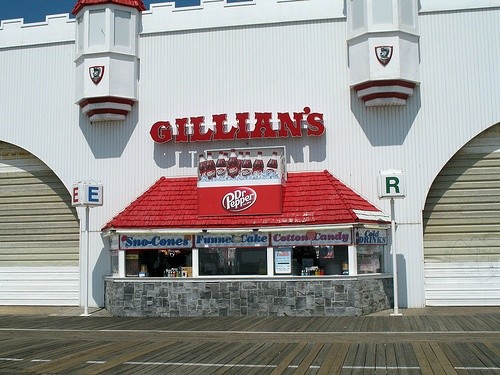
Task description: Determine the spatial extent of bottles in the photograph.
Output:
[224,153,228,165]
[301,268,324,276]
[266,152,278,173]
[160,269,185,277]
[226,149,238,177]
[253,152,264,175]
[216,151,226,179]
[237,152,243,173]
[241,152,252,178]
[199,154,207,180]
[207,152,216,180]
